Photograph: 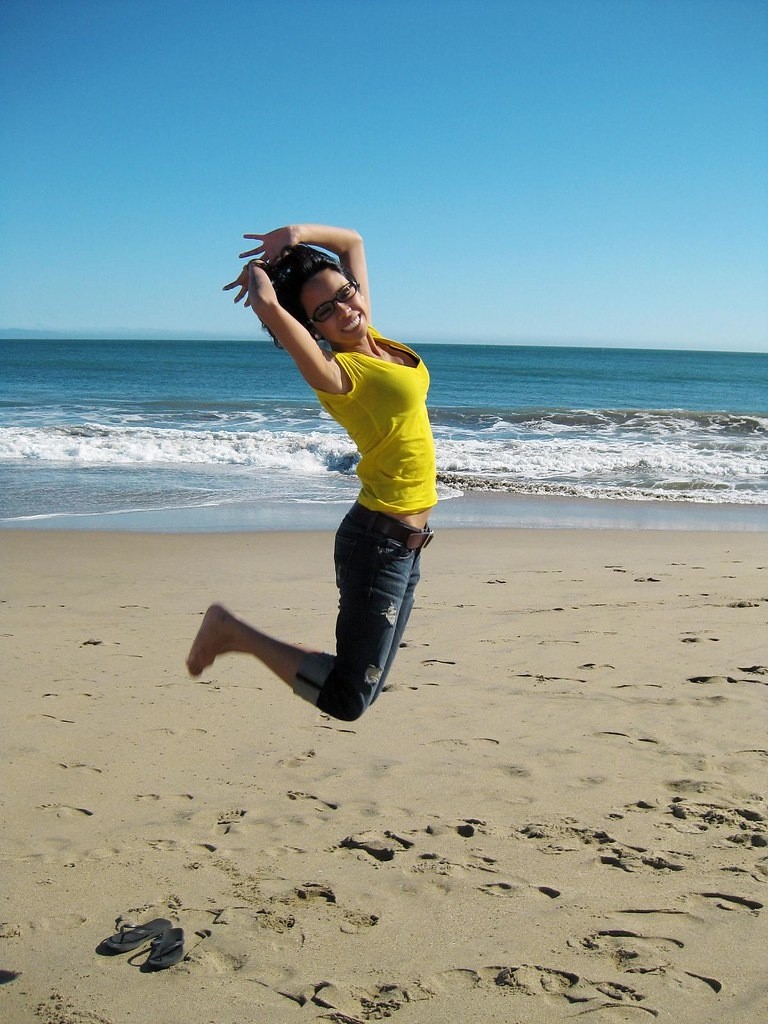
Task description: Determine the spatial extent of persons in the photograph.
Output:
[186,226,437,721]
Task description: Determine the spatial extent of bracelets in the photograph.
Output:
[247,259,268,271]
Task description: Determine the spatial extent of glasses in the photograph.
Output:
[309,278,359,326]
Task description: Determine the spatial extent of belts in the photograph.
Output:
[349,501,435,549]
